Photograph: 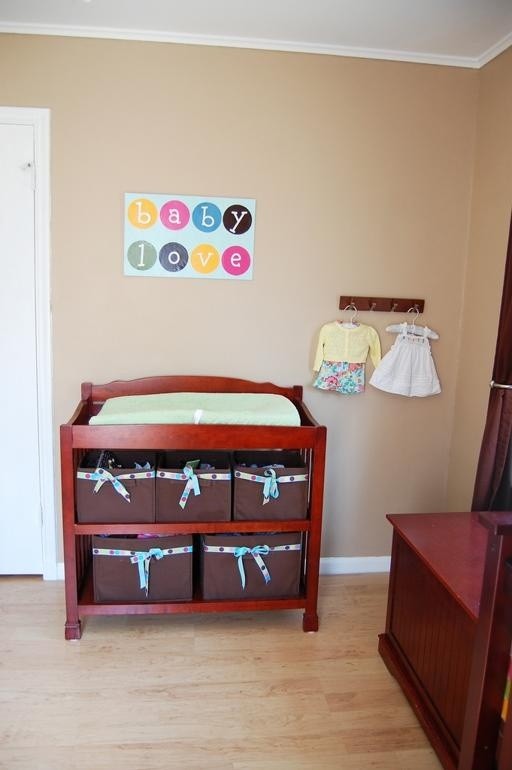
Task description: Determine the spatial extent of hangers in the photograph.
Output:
[336,304,361,330]
[385,307,439,340]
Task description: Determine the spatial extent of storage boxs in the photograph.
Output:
[156,452,233,524]
[75,450,156,524]
[198,533,305,602]
[89,533,194,605]
[233,450,310,522]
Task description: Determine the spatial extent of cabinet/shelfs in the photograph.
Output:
[59,374,328,640]
